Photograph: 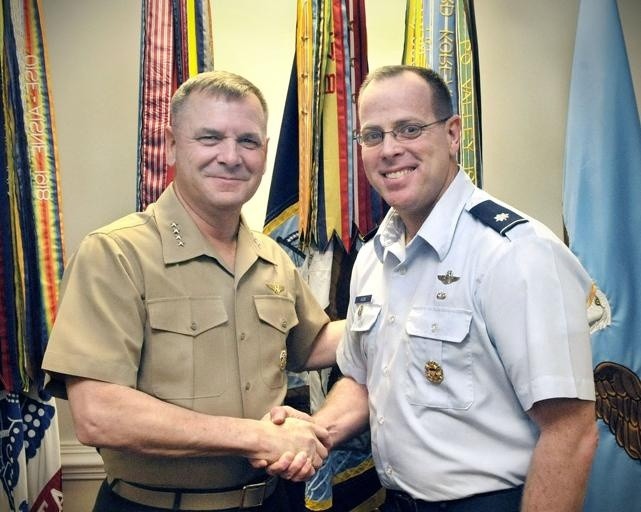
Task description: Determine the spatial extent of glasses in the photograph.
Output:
[353,117,448,147]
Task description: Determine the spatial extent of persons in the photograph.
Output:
[38,67,350,512]
[246,64,600,512]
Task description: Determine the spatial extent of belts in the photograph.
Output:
[386,489,449,512]
[107,475,279,510]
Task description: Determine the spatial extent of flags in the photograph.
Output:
[136,0,214,213]
[561,1,640,511]
[0,0,68,512]
[400,1,485,189]
[258,1,390,512]
